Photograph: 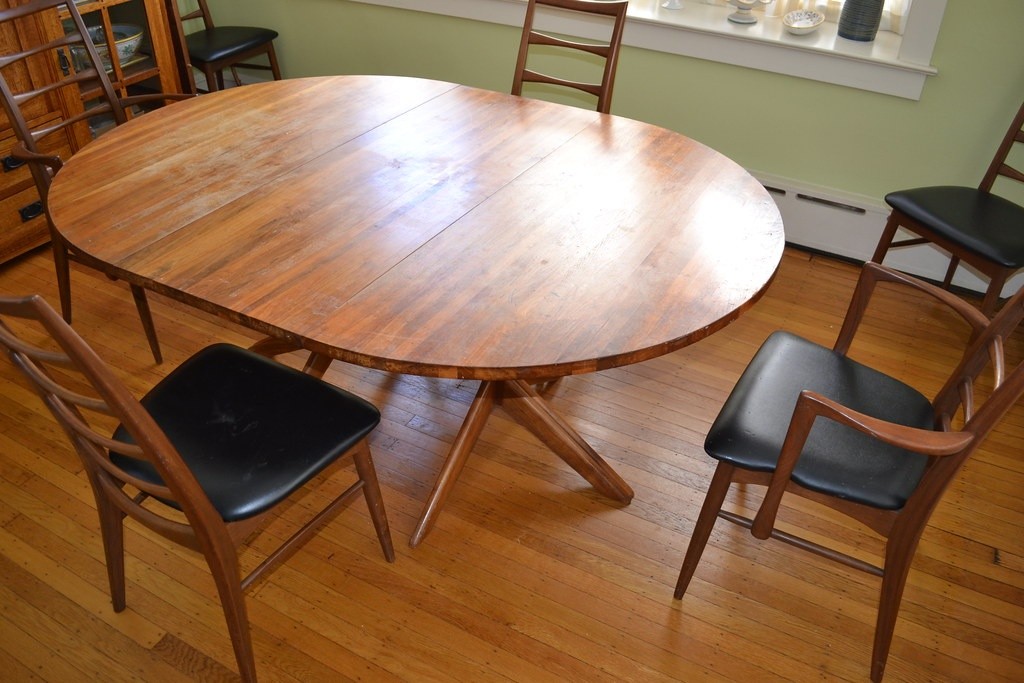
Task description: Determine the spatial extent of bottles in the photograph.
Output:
[838,0,884,41]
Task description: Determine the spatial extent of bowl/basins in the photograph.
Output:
[783,9,825,35]
[66,23,143,69]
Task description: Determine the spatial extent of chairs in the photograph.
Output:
[668,260,1024,683]
[0,0,202,367]
[177,0,285,94]
[0,291,397,683]
[871,100,1024,358]
[511,0,630,116]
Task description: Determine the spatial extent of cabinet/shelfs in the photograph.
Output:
[0,0,198,270]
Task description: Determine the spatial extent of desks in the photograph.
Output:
[49,74,788,553]
